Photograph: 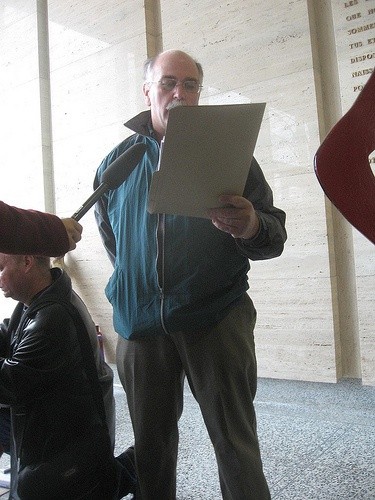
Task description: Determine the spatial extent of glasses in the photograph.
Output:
[145,79,203,94]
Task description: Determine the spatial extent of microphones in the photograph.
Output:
[70,142,147,222]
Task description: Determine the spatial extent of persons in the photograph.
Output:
[0,200,83,256]
[0,252,137,500]
[92,49,288,500]
